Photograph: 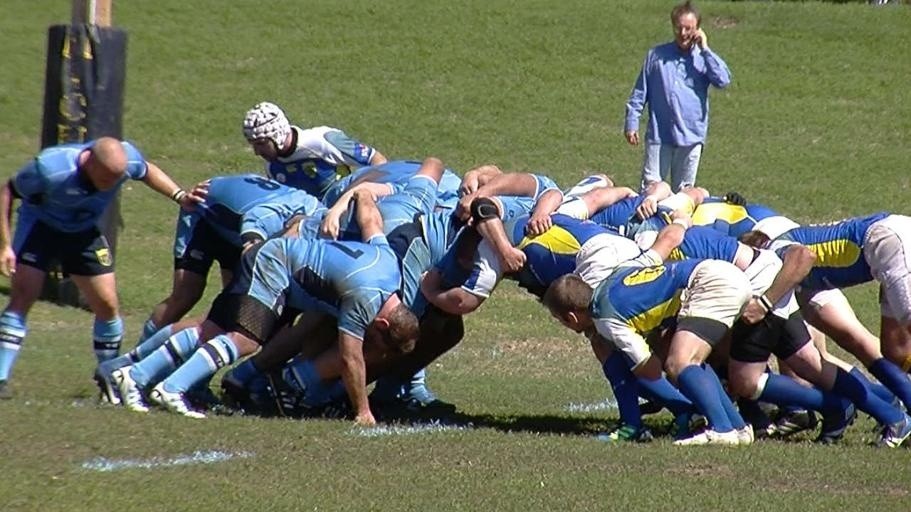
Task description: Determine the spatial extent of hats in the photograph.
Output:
[242,102,291,150]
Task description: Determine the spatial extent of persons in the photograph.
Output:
[93,157,910,448]
[242,101,388,201]
[624,4,733,194]
[0,136,211,404]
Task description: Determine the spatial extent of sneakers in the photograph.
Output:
[0,379,12,399]
